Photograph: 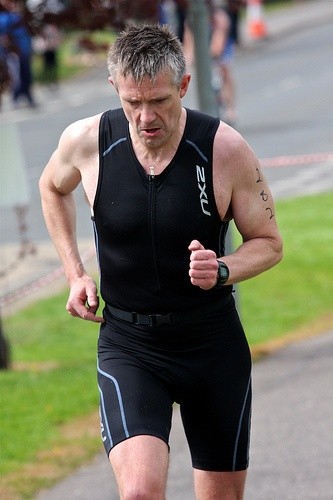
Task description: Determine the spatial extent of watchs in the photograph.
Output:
[216,260,230,286]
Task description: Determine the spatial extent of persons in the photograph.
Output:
[39,22,283,500]
